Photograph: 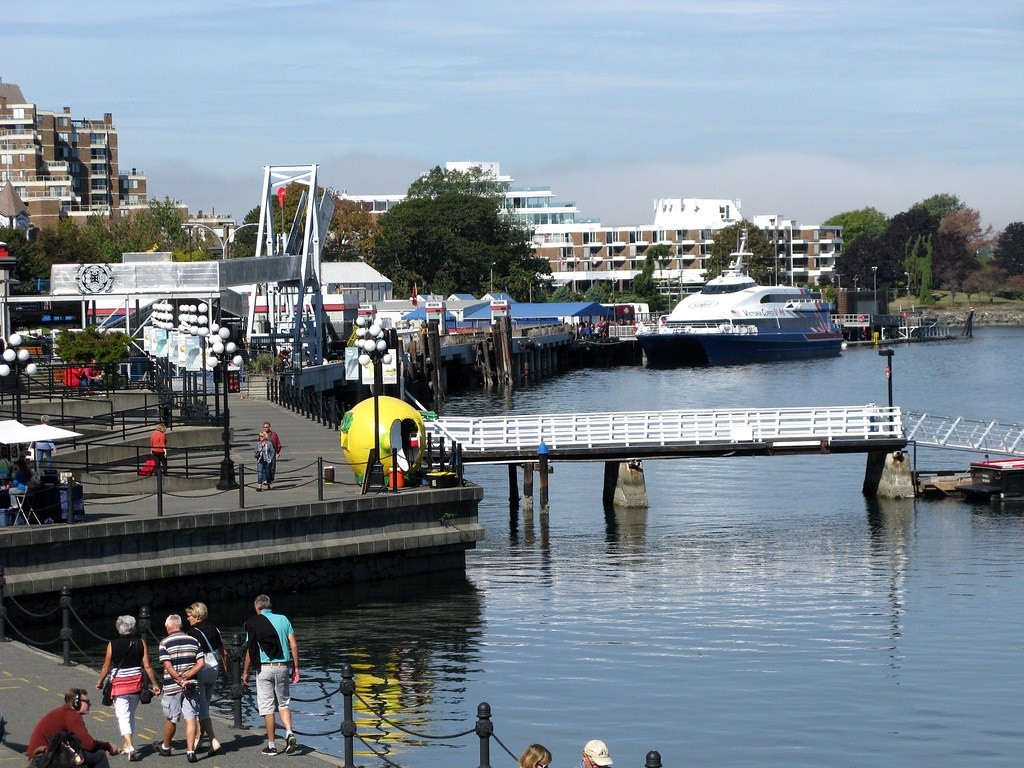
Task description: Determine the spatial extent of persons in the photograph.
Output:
[255,431,275,490]
[78,358,102,395]
[151,614,206,763]
[28,689,122,768]
[29,730,85,768]
[185,602,226,755]
[520,743,552,768]
[243,595,299,755]
[28,415,57,470]
[95,615,161,759]
[578,319,632,341]
[150,423,168,475]
[576,740,615,768]
[263,422,282,484]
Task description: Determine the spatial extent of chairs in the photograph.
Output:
[12,481,41,526]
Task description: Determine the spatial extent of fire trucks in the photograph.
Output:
[64,355,158,392]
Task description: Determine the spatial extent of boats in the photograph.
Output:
[574,333,639,368]
[954,453,1024,513]
[634,221,843,364]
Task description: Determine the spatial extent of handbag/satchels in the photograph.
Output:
[102,678,113,706]
[140,687,151,704]
[254,451,260,458]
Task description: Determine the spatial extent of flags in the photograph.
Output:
[278,187,285,207]
[412,284,417,306]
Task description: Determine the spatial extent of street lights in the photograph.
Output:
[178,302,223,425]
[151,296,173,387]
[878,348,894,433]
[354,313,394,492]
[181,223,265,261]
[206,326,244,489]
[904,271,909,312]
[871,266,878,313]
[0,333,37,423]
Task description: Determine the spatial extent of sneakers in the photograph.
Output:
[261,746,277,756]
[284,734,296,754]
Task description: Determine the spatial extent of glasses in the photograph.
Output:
[80,700,88,704]
[537,763,548,768]
[188,606,193,610]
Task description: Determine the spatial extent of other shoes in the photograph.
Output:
[122,744,136,761]
[9,505,18,509]
[159,741,171,756]
[194,740,203,750]
[187,753,197,762]
[256,487,261,492]
[207,738,221,755]
[267,484,272,490]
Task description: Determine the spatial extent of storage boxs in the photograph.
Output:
[426,472,458,489]
[0,508,26,526]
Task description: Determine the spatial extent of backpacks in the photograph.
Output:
[38,732,88,768]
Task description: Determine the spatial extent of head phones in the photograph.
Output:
[72,689,81,710]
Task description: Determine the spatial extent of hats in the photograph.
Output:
[584,740,613,766]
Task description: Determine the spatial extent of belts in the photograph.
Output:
[260,663,289,666]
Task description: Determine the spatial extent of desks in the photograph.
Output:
[48,484,84,523]
